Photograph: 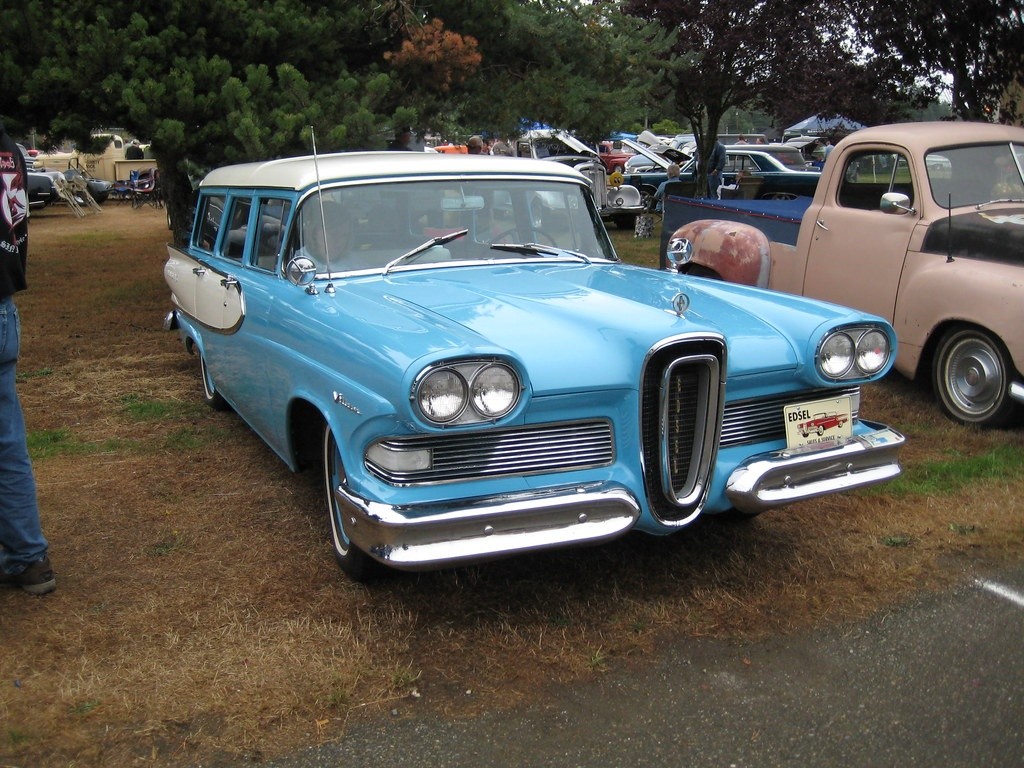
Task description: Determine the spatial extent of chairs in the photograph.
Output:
[721,176,764,201]
[649,181,695,225]
[113,170,141,206]
[130,167,160,208]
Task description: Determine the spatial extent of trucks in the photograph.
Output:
[32,132,159,207]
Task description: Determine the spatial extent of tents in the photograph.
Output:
[784,110,876,183]
[483,118,639,158]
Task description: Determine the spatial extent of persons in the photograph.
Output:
[466,136,483,154]
[125,139,144,159]
[0,124,56,596]
[716,170,753,201]
[389,124,413,151]
[755,137,765,144]
[734,135,749,145]
[653,165,682,212]
[815,140,834,162]
[482,139,495,155]
[692,136,726,200]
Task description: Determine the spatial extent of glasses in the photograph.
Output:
[466,142,480,150]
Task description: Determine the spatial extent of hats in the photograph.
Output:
[132,139,141,144]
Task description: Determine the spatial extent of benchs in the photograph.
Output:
[234,246,451,273]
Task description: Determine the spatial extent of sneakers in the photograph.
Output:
[1,555,59,597]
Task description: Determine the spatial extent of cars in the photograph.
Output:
[424,132,825,231]
[923,154,951,172]
[161,125,907,587]
[14,141,113,208]
[659,122,1023,428]
